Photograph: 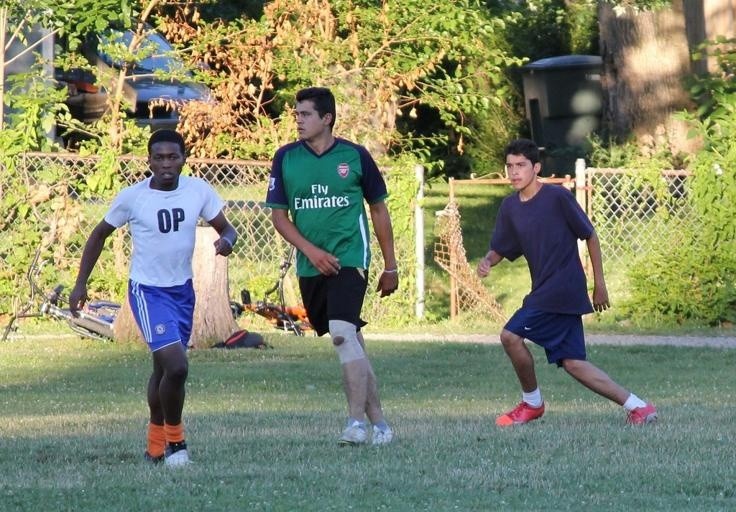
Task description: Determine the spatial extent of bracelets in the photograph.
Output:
[384,269,397,272]
[222,234,233,250]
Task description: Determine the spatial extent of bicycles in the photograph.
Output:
[229,247,314,337]
[1,249,121,344]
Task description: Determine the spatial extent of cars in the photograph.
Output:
[54,16,218,143]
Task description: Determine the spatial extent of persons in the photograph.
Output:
[266,87,398,446]
[478,140,658,430]
[68,129,236,469]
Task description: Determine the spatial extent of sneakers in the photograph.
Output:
[337,415,369,445]
[373,426,395,444]
[624,400,660,426]
[142,450,163,466]
[494,399,546,427]
[161,438,190,469]
